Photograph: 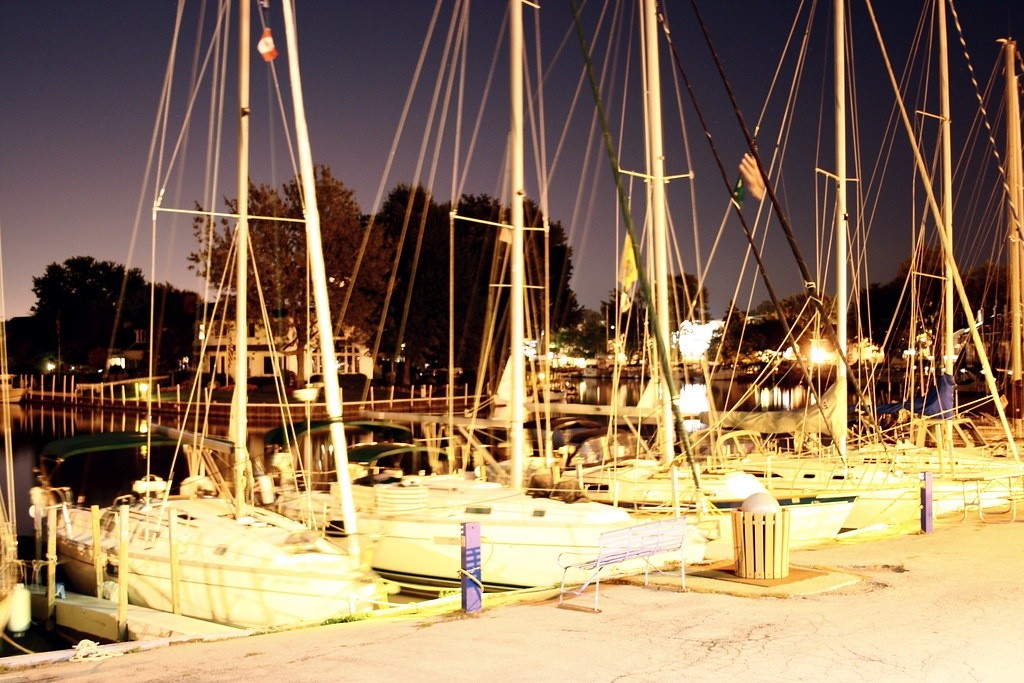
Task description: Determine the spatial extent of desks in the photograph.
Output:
[952,471,1024,522]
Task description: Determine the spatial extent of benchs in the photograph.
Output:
[558,517,686,612]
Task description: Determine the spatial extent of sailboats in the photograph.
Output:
[1,1,1024,649]
